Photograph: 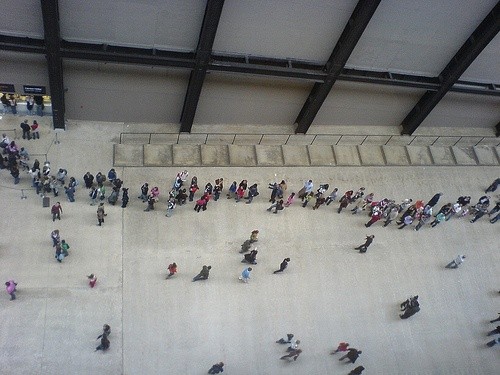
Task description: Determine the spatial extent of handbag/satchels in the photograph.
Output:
[14,282,18,286]
[66,243,70,249]
[276,203,284,210]
[360,245,367,252]
[253,187,259,196]
[357,206,362,213]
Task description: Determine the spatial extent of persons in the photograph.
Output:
[399,295,420,319]
[487,313,500,347]
[51,202,63,222]
[0,132,76,202]
[20,119,39,140]
[354,234,375,251]
[25,95,45,116]
[334,342,365,375]
[138,169,500,231]
[88,273,97,288]
[4,279,16,300]
[51,229,69,263]
[276,333,302,361]
[165,231,291,283]
[95,323,111,351]
[445,254,466,268]
[84,168,129,208]
[208,361,224,375]
[96,203,104,226]
[0,94,17,114]
